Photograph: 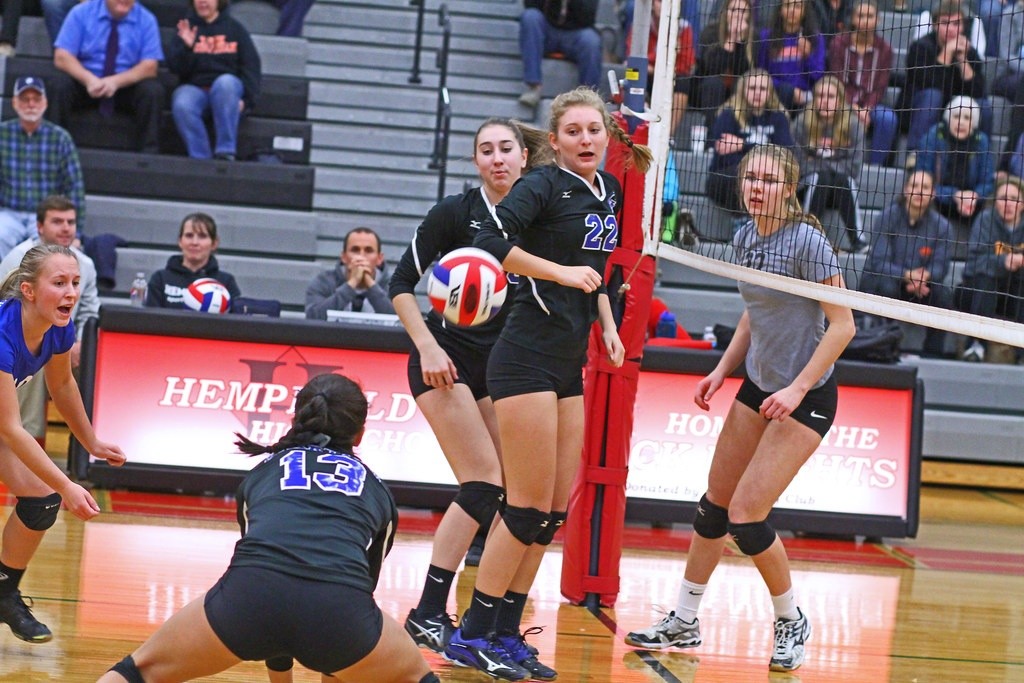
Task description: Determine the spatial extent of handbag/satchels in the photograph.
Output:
[822,321,903,363]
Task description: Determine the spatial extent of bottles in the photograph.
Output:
[129,272,148,310]
[656,312,676,337]
[703,326,717,349]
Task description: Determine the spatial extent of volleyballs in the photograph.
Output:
[426,248,509,330]
[183,278,231,315]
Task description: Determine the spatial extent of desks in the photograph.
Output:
[66,305,924,544]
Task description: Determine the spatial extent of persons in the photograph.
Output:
[304,228,398,322]
[0,244,125,642]
[518,0,1023,367]
[0,75,85,288]
[388,117,559,669]
[444,88,654,683]
[95,373,475,682]
[0,196,100,451]
[144,211,243,314]
[622,144,859,673]
[0,0,316,162]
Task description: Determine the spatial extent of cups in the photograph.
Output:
[690,125,708,153]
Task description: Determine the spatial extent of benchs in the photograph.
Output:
[0,0,1024,351]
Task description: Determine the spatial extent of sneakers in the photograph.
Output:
[0,590,52,642]
[626,610,702,648]
[404,607,459,652]
[498,627,558,681]
[769,606,813,673]
[442,628,533,682]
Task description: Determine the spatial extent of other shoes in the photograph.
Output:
[964,345,984,361]
[519,90,540,106]
[907,152,916,169]
[851,241,872,253]
[464,546,482,565]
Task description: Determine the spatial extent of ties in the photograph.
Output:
[104,22,119,75]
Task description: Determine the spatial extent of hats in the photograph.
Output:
[13,76,46,96]
